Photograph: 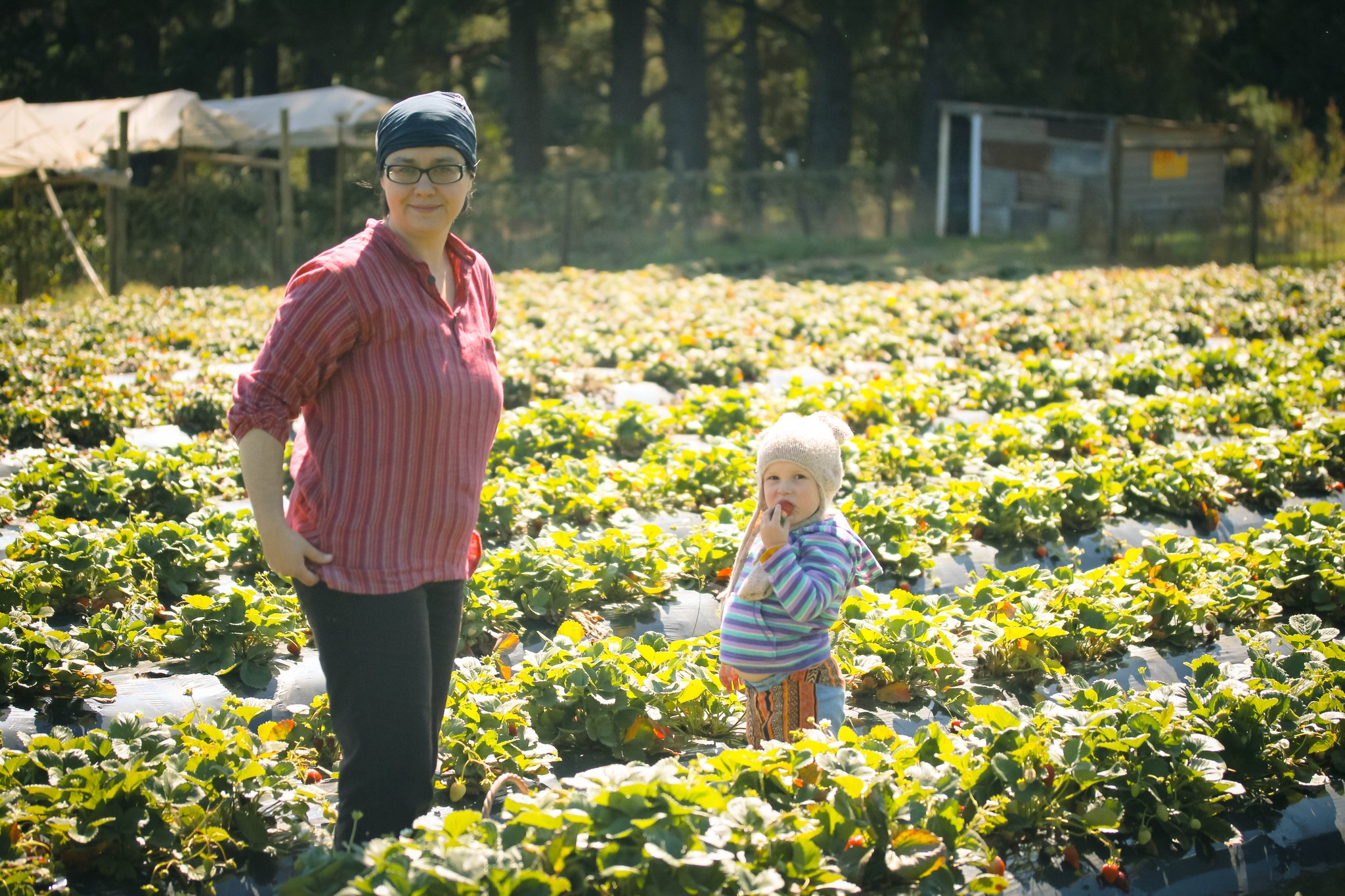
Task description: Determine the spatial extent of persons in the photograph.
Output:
[230,91,504,852]
[718,409,887,751]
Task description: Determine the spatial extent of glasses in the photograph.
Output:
[380,162,470,184]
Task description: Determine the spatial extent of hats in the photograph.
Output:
[720,408,858,596]
